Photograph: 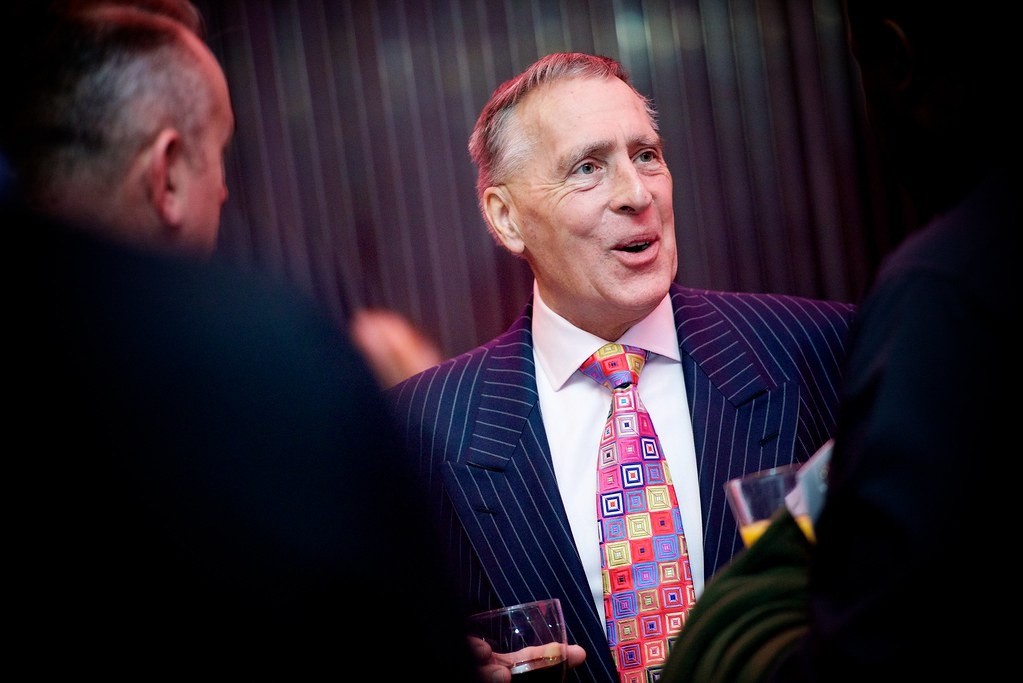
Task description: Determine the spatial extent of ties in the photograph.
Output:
[578,342,697,682]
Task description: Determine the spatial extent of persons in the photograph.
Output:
[0,208,486,682]
[380,54,855,683]
[0,0,234,262]
[655,188,1023,682]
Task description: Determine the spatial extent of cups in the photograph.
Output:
[724,463,816,550]
[460,598,568,683]
